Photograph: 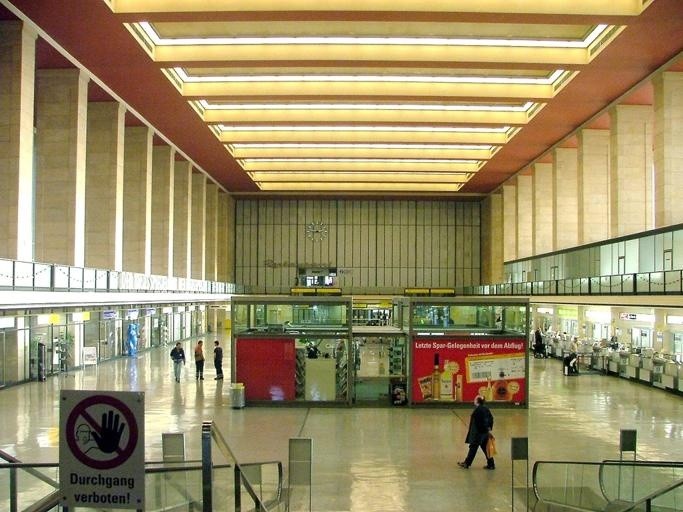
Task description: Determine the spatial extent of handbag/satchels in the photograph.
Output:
[486,437,499,458]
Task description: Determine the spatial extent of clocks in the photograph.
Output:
[306,221,328,242]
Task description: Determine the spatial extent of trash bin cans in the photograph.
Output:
[230,386,246,409]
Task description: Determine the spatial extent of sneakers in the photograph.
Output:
[483,465,495,469]
[456,461,469,468]
[176,377,223,383]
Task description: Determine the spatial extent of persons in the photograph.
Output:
[193,340,205,380]
[456,395,496,470]
[170,341,185,384]
[335,337,345,351]
[304,344,318,359]
[213,340,224,380]
[534,327,547,358]
[568,336,579,374]
[331,345,343,384]
